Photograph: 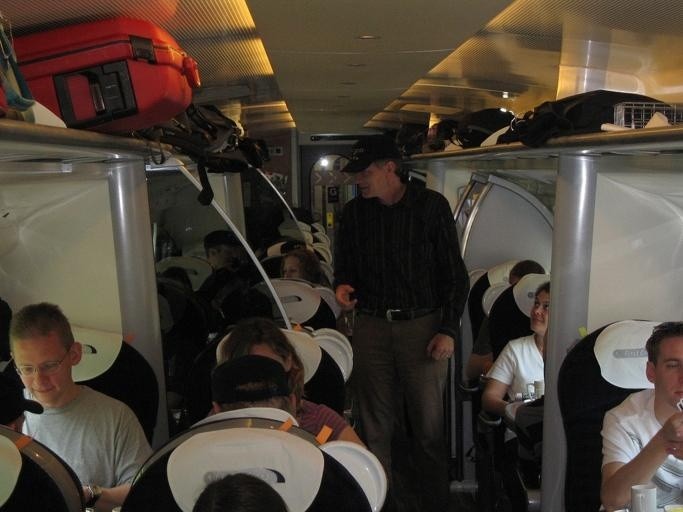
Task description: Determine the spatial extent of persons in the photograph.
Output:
[1,229,551,511]
[329,137,468,512]
[600,322,683,511]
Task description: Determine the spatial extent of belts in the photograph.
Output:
[360,307,435,323]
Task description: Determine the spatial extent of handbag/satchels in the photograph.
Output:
[477,435,512,472]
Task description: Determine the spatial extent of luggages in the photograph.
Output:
[1,18,200,132]
[479,435,526,512]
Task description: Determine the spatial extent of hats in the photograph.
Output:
[208,287,272,346]
[212,354,289,403]
[341,135,398,172]
[204,230,239,247]
[1,373,43,425]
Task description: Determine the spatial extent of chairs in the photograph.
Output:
[0,223,663,511]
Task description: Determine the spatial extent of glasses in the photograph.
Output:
[12,353,68,375]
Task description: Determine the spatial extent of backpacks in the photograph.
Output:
[515,90,679,148]
[140,102,270,172]
[397,109,515,154]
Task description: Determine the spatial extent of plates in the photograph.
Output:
[664,504,683,512]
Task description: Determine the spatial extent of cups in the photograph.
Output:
[630,484,658,512]
[525,381,544,401]
